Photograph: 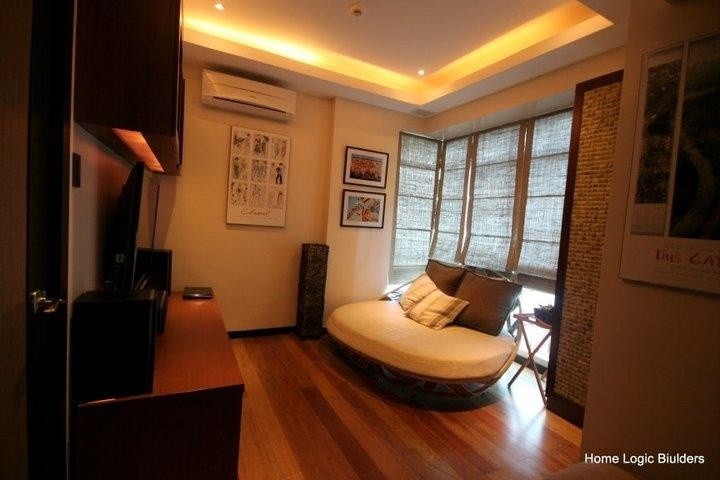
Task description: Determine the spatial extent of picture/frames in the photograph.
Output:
[342,143,391,191]
[340,187,387,230]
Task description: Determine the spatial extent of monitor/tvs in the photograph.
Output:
[102,160,160,289]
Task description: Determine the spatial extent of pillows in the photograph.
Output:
[406,289,468,333]
[452,267,522,336]
[425,259,465,299]
[398,272,435,309]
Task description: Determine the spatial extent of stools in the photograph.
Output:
[507,306,556,407]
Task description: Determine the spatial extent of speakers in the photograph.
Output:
[73,289,157,394]
[152,249,172,296]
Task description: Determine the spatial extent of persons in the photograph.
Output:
[231,134,286,209]
[351,156,381,180]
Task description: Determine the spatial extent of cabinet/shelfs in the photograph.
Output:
[73,0,187,179]
[72,282,249,479]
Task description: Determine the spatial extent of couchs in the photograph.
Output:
[324,250,526,405]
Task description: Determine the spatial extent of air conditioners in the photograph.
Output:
[199,66,298,123]
